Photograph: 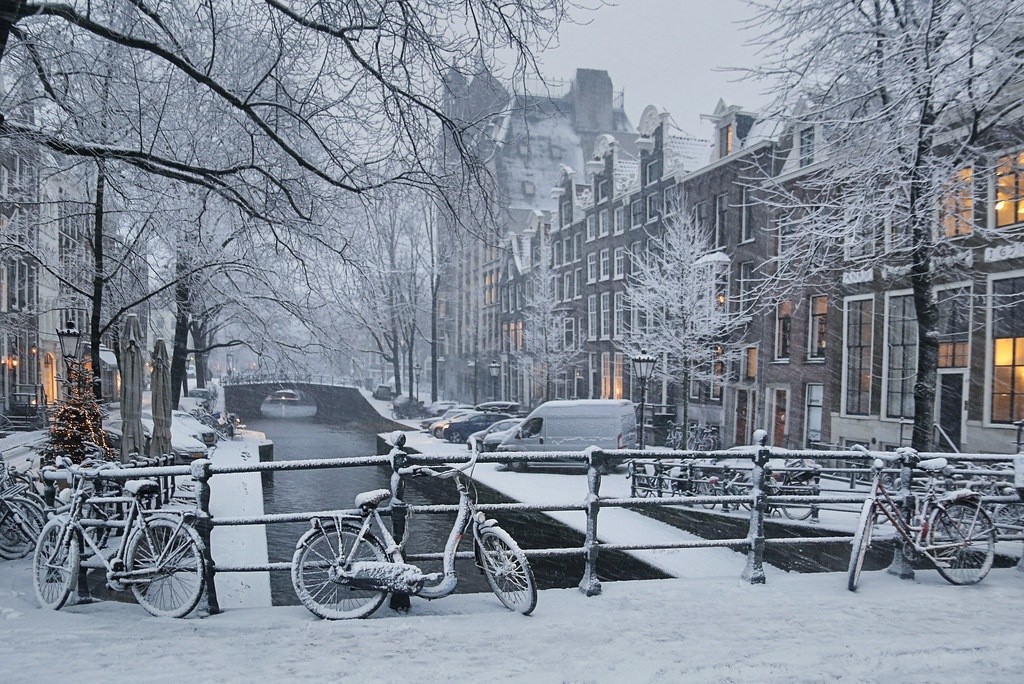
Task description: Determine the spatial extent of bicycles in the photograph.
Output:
[31,456,207,619]
[391,401,434,420]
[290,433,538,621]
[633,458,1024,537]
[0,437,109,560]
[846,443,999,591]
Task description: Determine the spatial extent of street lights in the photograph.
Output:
[487,359,502,402]
[632,348,658,450]
[413,362,422,401]
[55,318,85,399]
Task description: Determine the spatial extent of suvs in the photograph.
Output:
[443,401,522,444]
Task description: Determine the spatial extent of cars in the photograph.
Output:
[392,393,417,408]
[693,444,815,483]
[420,400,475,438]
[482,421,521,452]
[467,417,527,453]
[373,384,395,400]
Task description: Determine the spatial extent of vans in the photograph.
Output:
[496,398,638,474]
